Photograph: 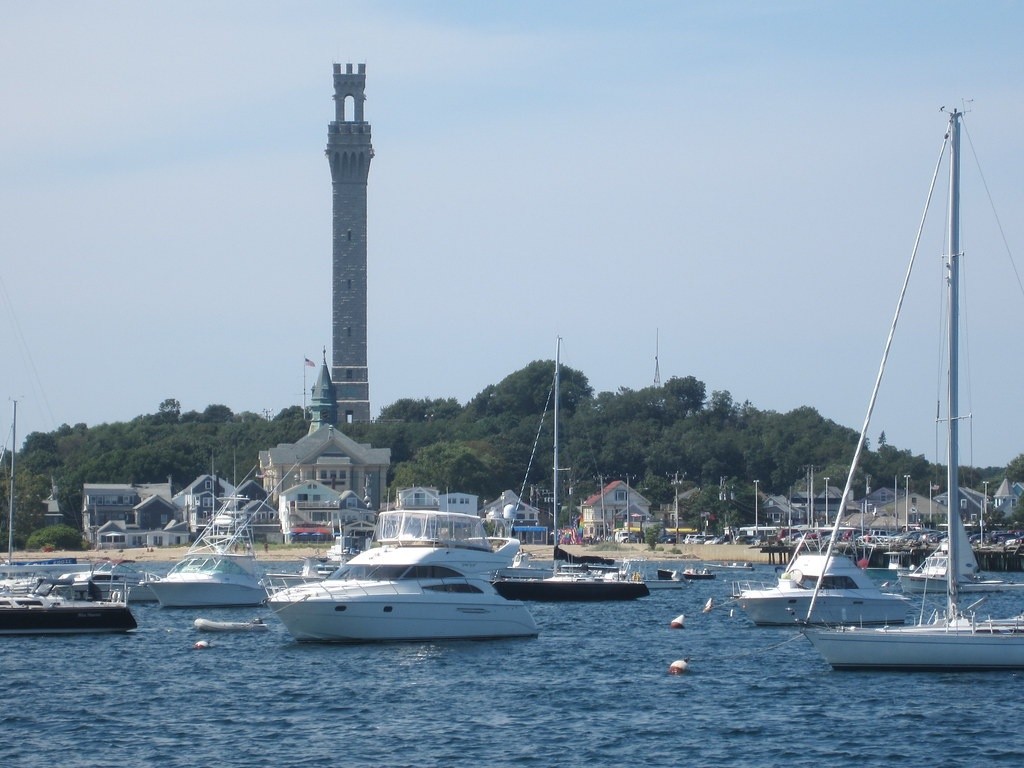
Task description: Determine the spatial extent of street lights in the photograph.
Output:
[983,481,989,514]
[823,477,829,526]
[903,474,911,532]
[753,480,759,537]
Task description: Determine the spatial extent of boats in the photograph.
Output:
[147,445,373,605]
[1,568,138,635]
[56,559,162,601]
[656,568,716,580]
[259,510,543,639]
[733,526,906,625]
[499,546,553,578]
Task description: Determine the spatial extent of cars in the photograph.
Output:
[608,526,1022,545]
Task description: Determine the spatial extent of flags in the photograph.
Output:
[305,358,315,368]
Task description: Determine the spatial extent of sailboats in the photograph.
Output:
[890,506,1023,591]
[0,397,103,576]
[492,334,650,597]
[791,103,1024,669]
